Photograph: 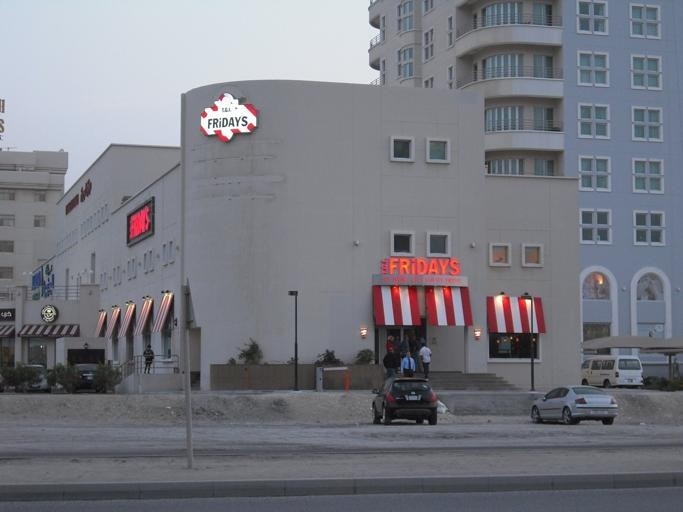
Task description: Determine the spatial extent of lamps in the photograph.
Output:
[359,327,367,338]
[473,328,480,340]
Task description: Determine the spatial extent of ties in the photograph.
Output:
[408,358,411,369]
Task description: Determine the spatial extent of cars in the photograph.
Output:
[533,380,617,424]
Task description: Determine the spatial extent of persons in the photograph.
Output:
[143,344,155,374]
[382,335,432,380]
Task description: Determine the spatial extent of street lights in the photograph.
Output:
[68,270,81,301]
[20,271,33,301]
[286,288,298,392]
[79,268,94,285]
[519,293,536,390]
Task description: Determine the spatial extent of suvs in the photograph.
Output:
[368,375,437,427]
[69,360,102,391]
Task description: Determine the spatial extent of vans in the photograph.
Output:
[580,356,643,390]
[17,364,49,395]
